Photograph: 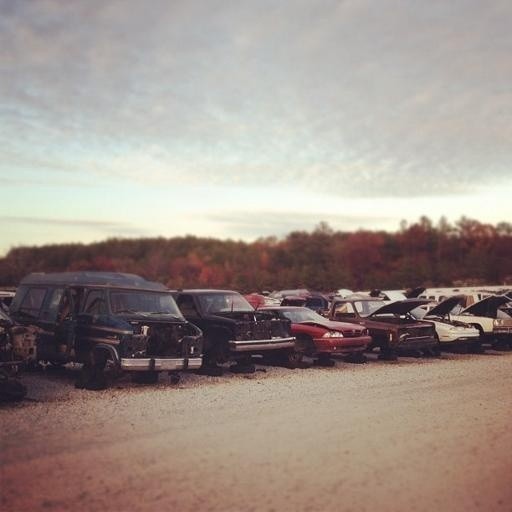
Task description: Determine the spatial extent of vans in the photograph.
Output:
[10,272,202,381]
